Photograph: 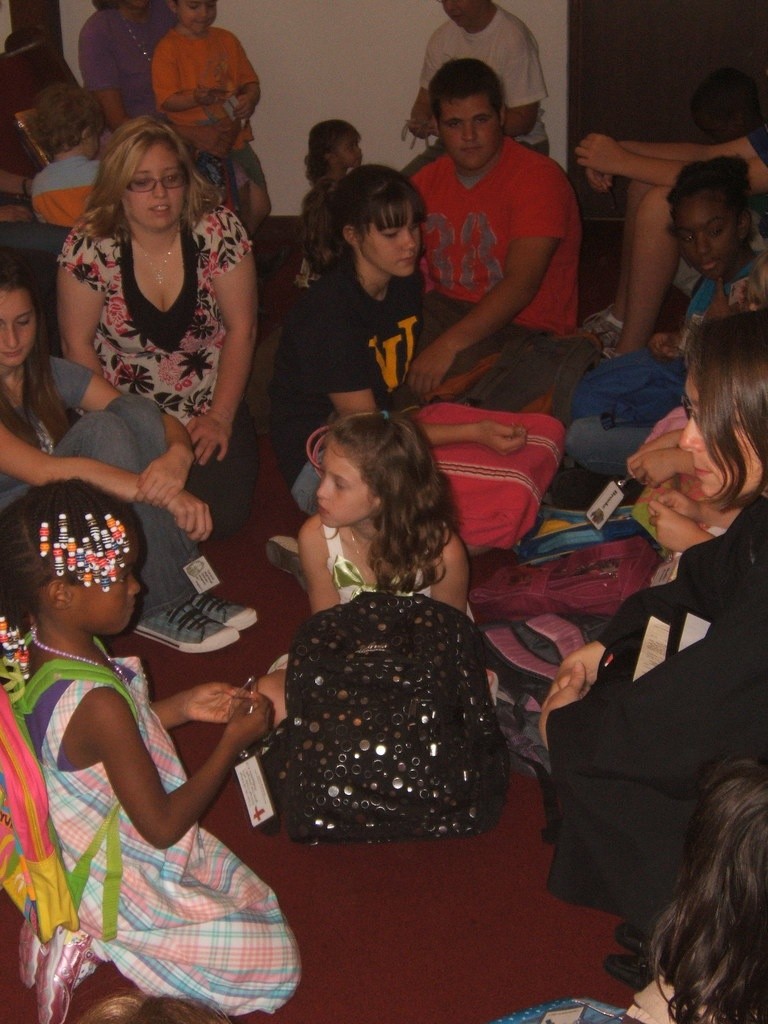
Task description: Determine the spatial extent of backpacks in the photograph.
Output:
[482,612,612,778]
[571,349,685,429]
[0,659,138,942]
[258,590,511,845]
[468,535,663,620]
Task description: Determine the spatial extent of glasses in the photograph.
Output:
[127,174,187,192]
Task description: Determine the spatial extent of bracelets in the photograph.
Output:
[22,176,29,200]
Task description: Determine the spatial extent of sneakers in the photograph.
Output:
[18,916,103,1024]
[189,592,257,630]
[133,601,240,653]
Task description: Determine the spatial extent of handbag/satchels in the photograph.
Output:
[306,403,566,551]
[389,290,598,423]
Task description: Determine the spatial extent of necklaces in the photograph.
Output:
[130,232,179,284]
[350,532,374,555]
[31,625,129,682]
[120,9,153,57]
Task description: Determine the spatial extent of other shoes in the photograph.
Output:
[603,922,650,989]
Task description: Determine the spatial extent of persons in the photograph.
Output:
[0,475,304,1024]
[557,66,768,566]
[29,82,106,230]
[285,119,372,320]
[250,409,481,730]
[618,750,768,1024]
[55,113,259,468]
[79,0,182,129]
[535,308,768,988]
[392,57,584,405]
[400,0,551,181]
[0,168,73,255]
[152,0,272,236]
[0,271,260,653]
[265,166,567,586]
